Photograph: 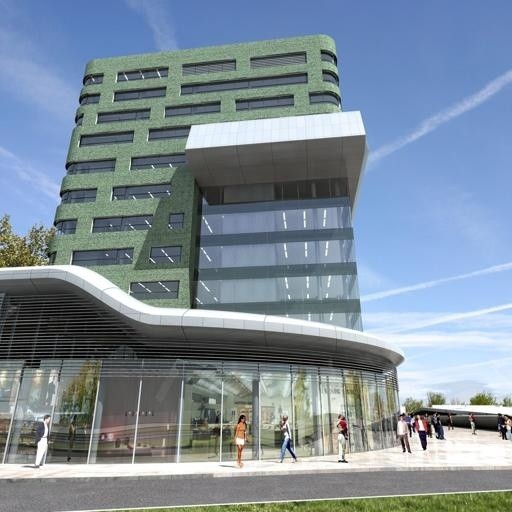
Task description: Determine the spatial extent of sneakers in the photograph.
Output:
[338,459,346,463]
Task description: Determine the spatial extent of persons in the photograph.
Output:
[276,413,298,463]
[416,413,428,450]
[234,414,248,466]
[35,414,52,469]
[336,414,348,463]
[66,415,77,462]
[401,411,455,440]
[396,414,412,453]
[497,413,511,441]
[336,415,348,439]
[468,413,477,435]
